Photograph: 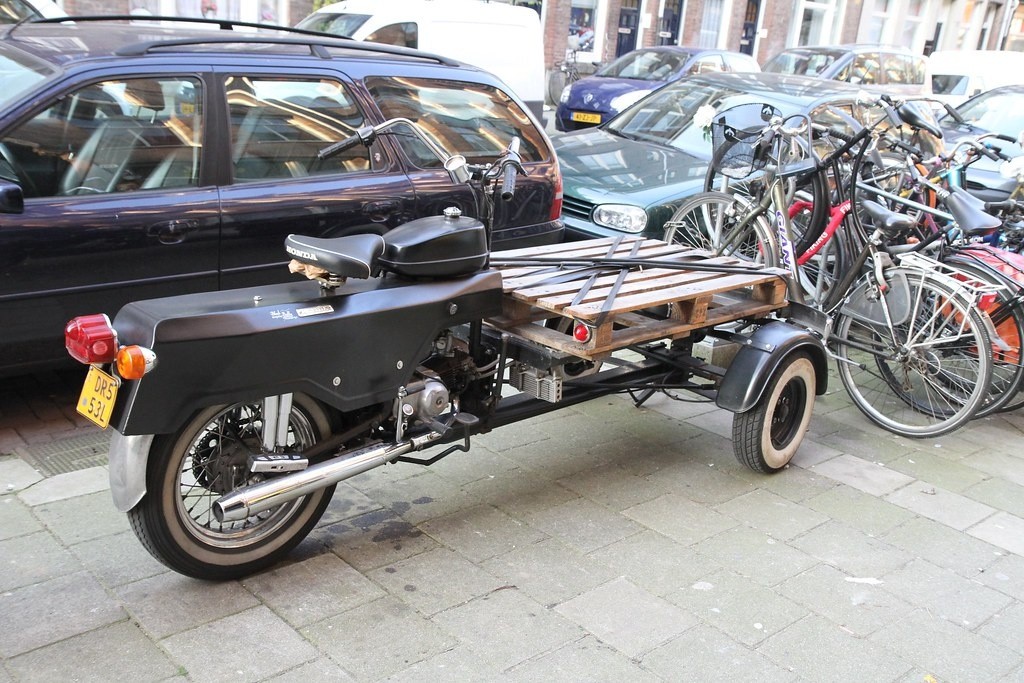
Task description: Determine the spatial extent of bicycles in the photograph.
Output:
[662,93,1024,440]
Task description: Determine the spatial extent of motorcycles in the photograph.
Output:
[64,117,831,584]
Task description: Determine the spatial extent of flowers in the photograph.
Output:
[692,105,718,143]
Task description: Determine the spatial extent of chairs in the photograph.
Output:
[60,78,166,191]
[141,102,274,187]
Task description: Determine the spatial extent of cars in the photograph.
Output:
[876,85,1023,195]
[555,45,762,132]
[760,45,935,98]
[549,72,945,245]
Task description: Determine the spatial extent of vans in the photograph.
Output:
[288,0,548,128]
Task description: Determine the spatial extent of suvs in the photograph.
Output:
[0,16,565,376]
